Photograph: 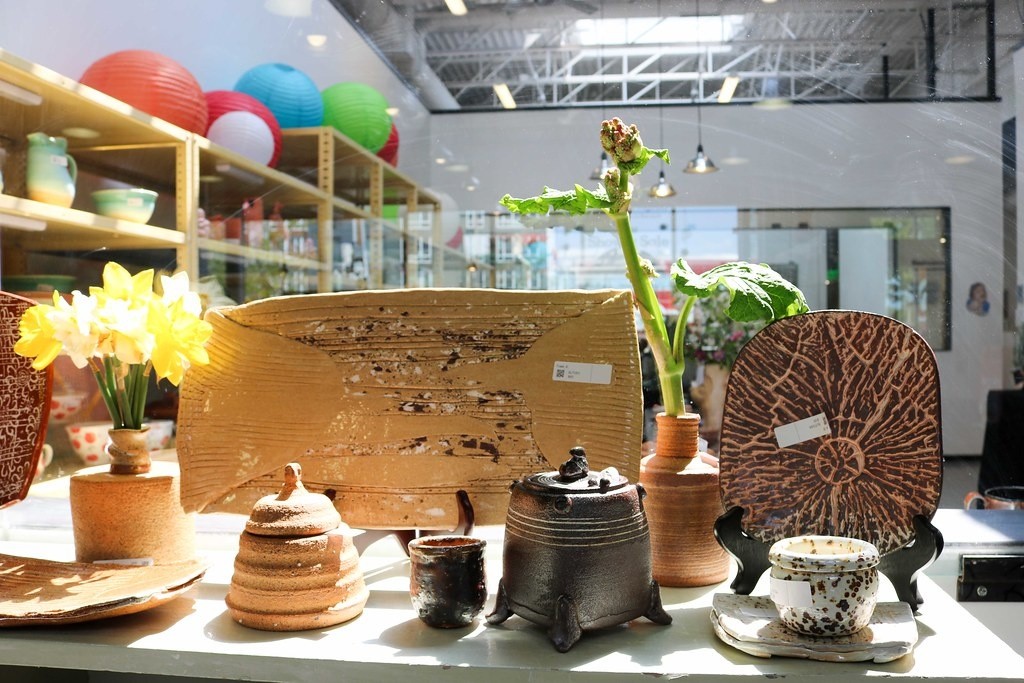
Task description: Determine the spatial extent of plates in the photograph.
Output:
[721,308,940,559]
[178,287,648,535]
[0,553,211,629]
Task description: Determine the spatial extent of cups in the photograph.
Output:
[406,534,488,631]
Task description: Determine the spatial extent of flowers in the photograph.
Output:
[638,314,696,406]
[684,314,754,370]
[14,261,213,428]
[498,118,812,415]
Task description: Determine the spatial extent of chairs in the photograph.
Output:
[977,389,1024,509]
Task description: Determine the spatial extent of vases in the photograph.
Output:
[653,404,693,449]
[69,427,198,565]
[698,362,735,434]
[636,410,730,588]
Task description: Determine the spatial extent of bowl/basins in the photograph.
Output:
[67,419,172,466]
[94,186,159,226]
[46,393,86,429]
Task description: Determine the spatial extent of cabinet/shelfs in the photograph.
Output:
[832,226,897,319]
[0,44,443,481]
[732,226,831,313]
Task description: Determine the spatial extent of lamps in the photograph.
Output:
[590,58,616,181]
[648,56,676,199]
[682,79,719,175]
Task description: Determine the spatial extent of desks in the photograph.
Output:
[0,460,1024,683]
